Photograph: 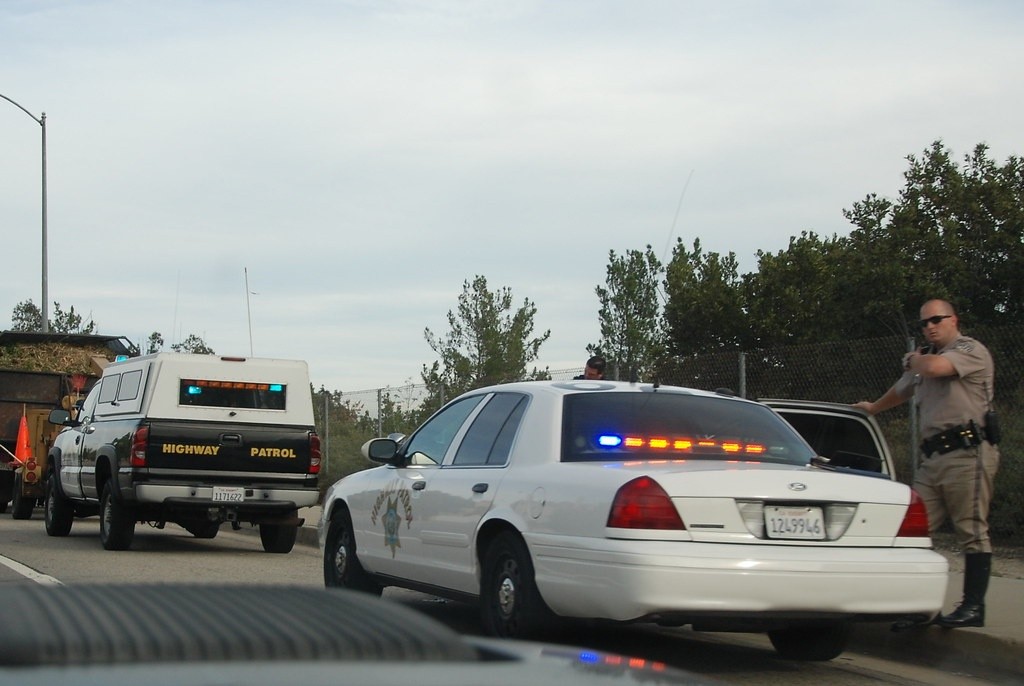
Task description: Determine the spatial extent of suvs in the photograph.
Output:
[39,351,326,554]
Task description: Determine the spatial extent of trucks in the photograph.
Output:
[0,330,143,522]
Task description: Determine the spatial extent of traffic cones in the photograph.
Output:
[11,415,33,468]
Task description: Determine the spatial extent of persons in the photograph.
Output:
[572,356,608,380]
[847,299,999,634]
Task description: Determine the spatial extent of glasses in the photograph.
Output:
[918,316,952,327]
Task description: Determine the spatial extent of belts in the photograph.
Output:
[919,427,988,457]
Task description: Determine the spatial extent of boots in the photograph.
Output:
[937,552,991,628]
[891,614,942,632]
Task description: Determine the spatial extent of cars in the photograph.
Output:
[317,379,951,663]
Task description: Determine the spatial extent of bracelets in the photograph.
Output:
[903,354,913,368]
